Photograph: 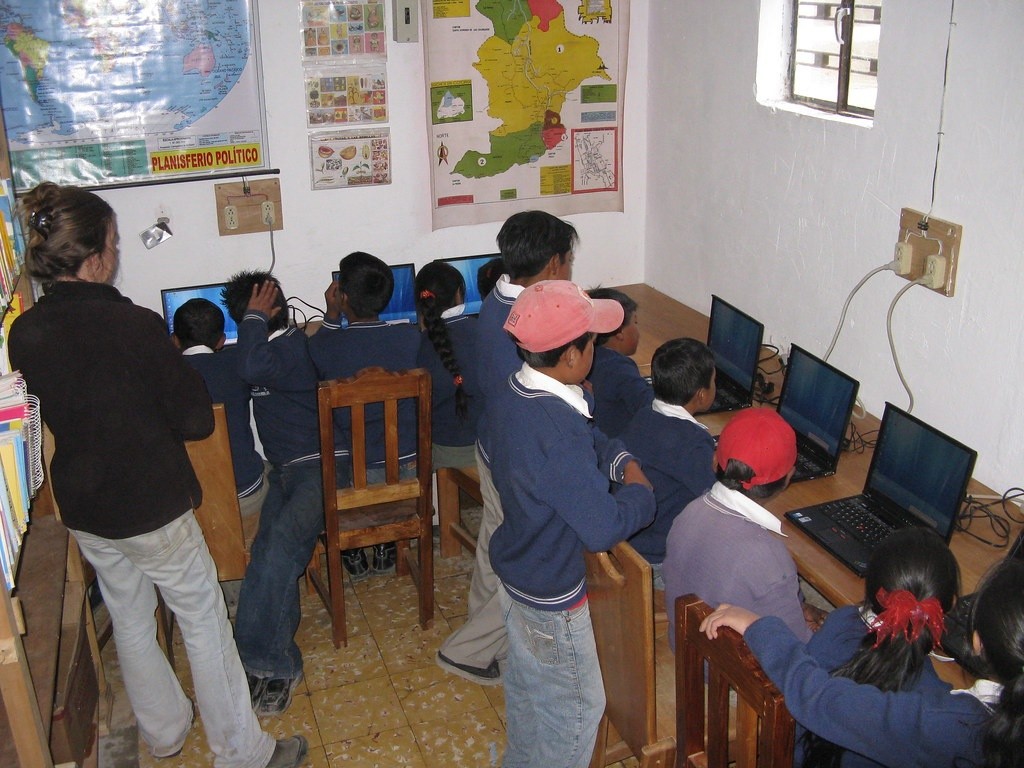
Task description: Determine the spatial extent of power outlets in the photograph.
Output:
[923,255,946,291]
[890,242,914,276]
[222,206,240,232]
[262,200,276,226]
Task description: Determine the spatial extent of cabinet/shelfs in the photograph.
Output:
[0,117,122,768]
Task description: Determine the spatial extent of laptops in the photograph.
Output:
[332,263,418,329]
[160,283,240,348]
[783,401,979,578]
[642,294,764,417]
[936,530,1024,683]
[434,253,504,319]
[712,342,860,485]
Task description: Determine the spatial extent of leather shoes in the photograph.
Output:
[245,669,304,716]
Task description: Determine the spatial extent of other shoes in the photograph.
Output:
[432,525,441,543]
[152,695,195,758]
[387,556,389,557]
[371,542,397,577]
[342,548,371,582]
[434,648,501,686]
[264,734,308,768]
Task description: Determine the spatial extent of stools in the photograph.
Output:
[439,464,484,556]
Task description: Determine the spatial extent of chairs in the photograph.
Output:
[314,367,438,654]
[586,507,682,767]
[155,402,261,666]
[671,590,801,767]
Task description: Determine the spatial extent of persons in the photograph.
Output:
[434,210,596,686]
[307,251,457,575]
[416,262,479,516]
[584,287,655,439]
[791,527,962,768]
[614,337,718,592]
[219,272,351,718]
[660,406,831,768]
[6,181,309,768]
[170,297,276,517]
[477,258,505,303]
[699,559,1024,768]
[487,279,657,768]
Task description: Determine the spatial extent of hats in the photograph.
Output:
[502,279,625,354]
[715,407,797,491]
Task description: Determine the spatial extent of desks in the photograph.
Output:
[297,283,1024,695]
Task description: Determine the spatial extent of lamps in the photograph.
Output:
[139,217,173,250]
